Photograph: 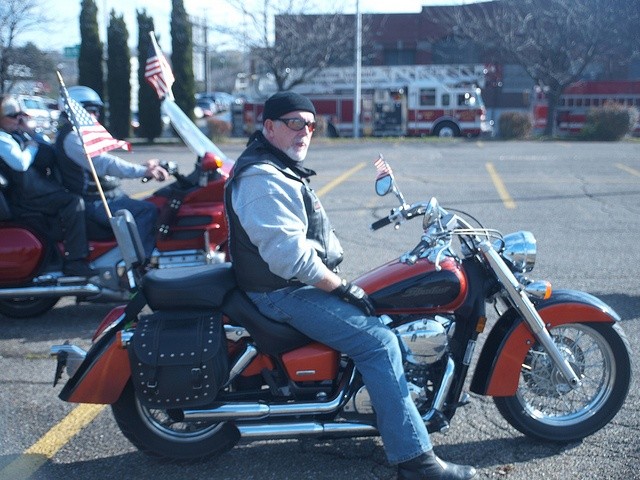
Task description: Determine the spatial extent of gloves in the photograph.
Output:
[328,277,376,317]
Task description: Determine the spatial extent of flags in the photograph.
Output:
[57,85,133,159]
[373,154,392,181]
[143,30,177,101]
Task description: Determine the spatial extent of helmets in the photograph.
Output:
[58,85,105,108]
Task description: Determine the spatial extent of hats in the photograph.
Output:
[263,92,316,123]
[1,97,30,119]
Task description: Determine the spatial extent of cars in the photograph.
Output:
[194,93,235,118]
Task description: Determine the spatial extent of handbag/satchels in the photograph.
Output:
[37,143,63,183]
[126,310,230,409]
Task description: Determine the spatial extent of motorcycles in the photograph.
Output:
[51,174,632,466]
[0,96,235,318]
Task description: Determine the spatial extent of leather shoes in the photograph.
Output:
[397,449,477,479]
[62,262,100,276]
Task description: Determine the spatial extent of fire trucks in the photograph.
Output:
[535,81,640,131]
[242,65,487,138]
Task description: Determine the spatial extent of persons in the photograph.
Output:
[0,95,101,277]
[326,114,340,138]
[54,84,167,294]
[223,89,478,480]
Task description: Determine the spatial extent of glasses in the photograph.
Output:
[276,118,316,131]
